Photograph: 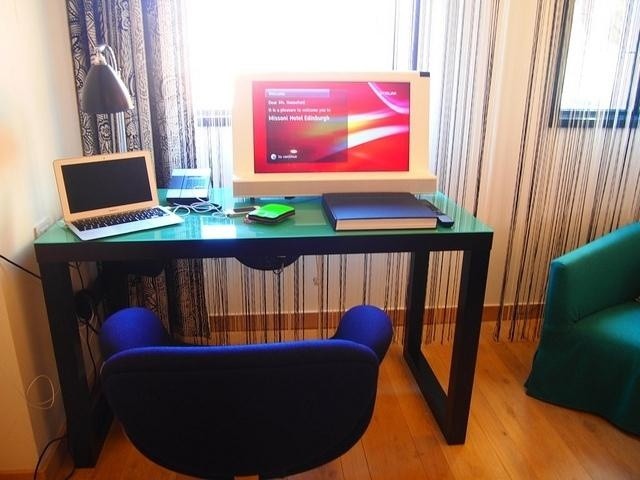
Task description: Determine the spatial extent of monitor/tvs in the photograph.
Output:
[232,71,437,213]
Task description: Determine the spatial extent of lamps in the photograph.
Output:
[81,43,134,153]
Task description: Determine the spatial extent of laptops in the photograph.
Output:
[53,149,185,242]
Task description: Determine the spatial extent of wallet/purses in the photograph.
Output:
[248,202,296,224]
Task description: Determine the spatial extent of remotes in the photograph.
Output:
[418,199,454,227]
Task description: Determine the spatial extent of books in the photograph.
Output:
[321,191,438,232]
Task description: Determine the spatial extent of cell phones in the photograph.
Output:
[223,205,260,218]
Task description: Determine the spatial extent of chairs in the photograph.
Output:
[98,304,393,480]
[524,220,640,435]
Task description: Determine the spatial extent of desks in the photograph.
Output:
[33,188,494,467]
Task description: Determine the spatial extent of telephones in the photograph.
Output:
[166,167,221,211]
